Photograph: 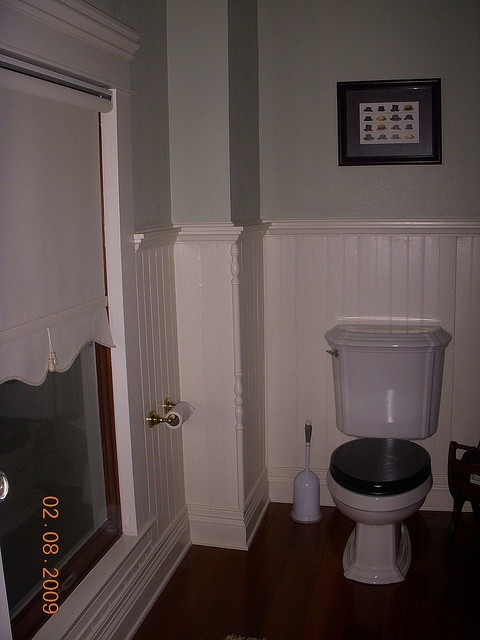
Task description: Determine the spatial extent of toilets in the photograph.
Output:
[324,324,452,583]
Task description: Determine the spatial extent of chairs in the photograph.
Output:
[444,441,480,543]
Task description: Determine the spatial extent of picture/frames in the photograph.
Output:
[336,78,443,165]
